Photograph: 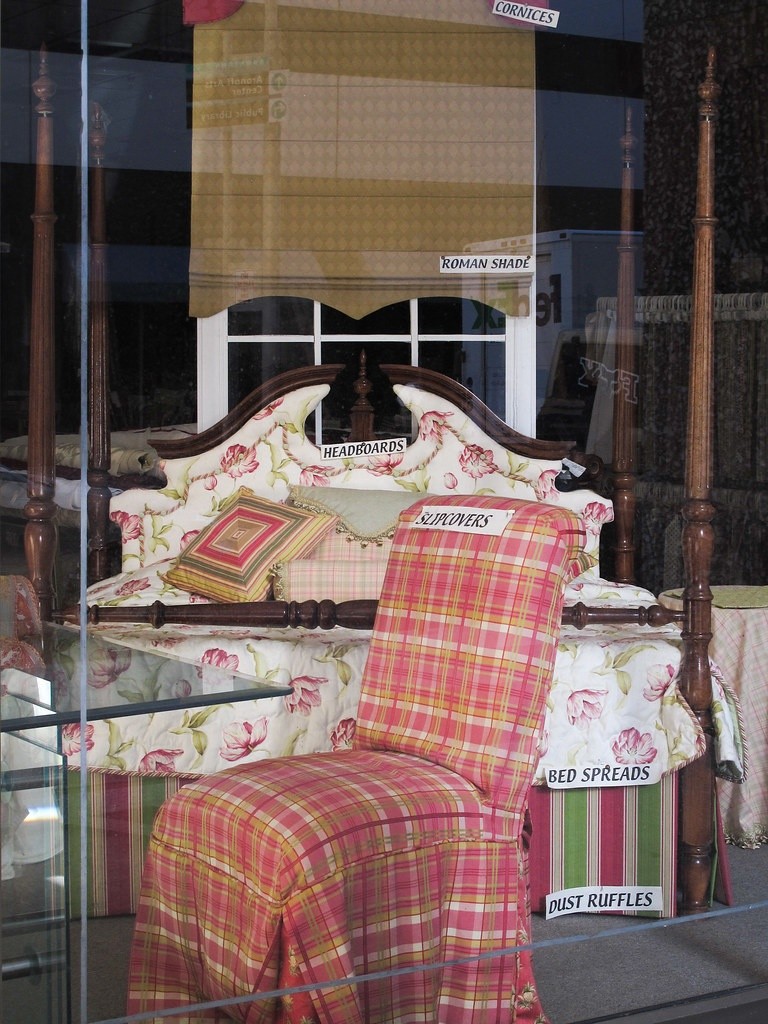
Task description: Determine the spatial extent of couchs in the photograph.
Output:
[22,43,726,921]
[1,422,195,538]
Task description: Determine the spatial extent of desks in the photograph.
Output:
[0,627,294,1024]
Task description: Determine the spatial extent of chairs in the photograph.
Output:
[125,494,599,1024]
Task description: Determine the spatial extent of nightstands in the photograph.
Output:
[658,583,768,851]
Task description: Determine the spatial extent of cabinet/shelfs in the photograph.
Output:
[463,230,644,439]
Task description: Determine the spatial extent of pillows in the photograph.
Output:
[267,484,436,602]
[161,484,341,602]
